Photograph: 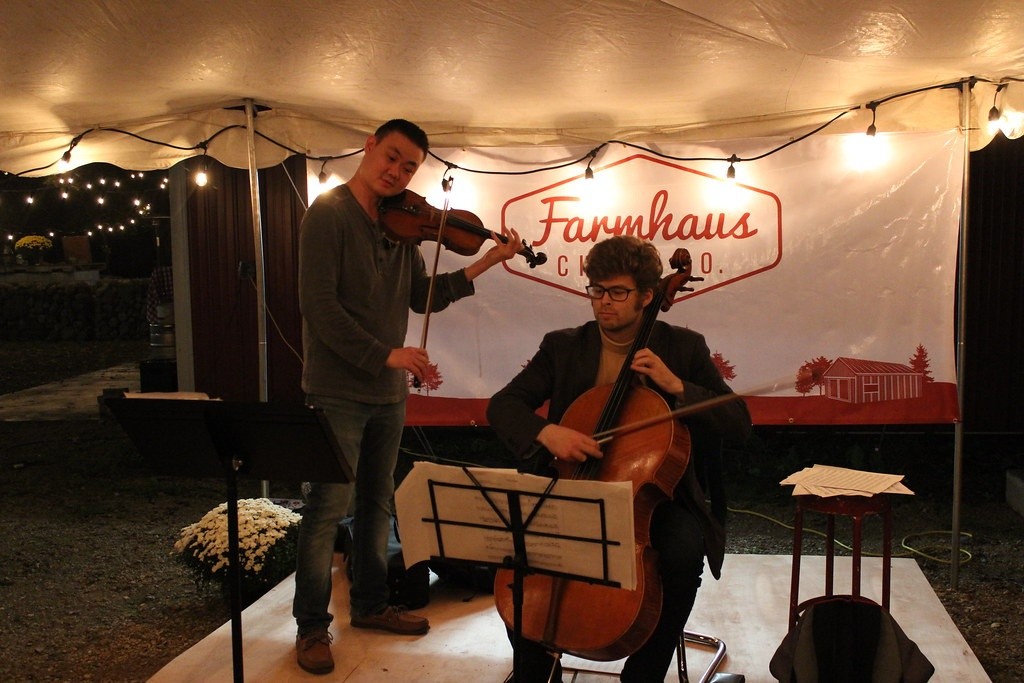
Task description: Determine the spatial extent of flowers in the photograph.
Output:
[13,236,52,257]
[171,498,302,600]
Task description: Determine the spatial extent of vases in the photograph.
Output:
[27,254,41,265]
[222,584,256,609]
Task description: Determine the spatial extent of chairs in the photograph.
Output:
[504,618,727,683]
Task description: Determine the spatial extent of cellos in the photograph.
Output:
[491,245,695,683]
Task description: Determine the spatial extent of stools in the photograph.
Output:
[788,489,894,633]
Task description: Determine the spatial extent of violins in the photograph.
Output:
[377,189,549,270]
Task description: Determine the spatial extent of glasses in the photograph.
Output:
[585,284,640,302]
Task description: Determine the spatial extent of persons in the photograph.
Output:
[293,118,525,674]
[484,235,752,683]
[146,253,174,323]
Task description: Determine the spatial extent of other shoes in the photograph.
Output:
[296,626,335,674]
[350,606,430,634]
